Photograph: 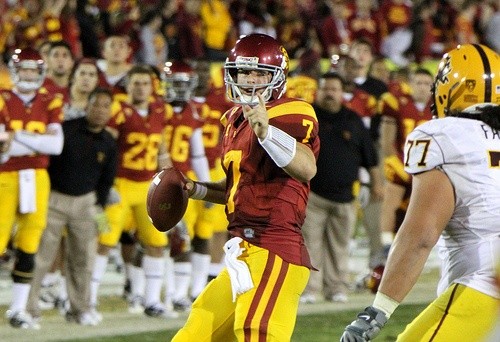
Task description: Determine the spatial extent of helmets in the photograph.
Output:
[223,33,289,105]
[160,61,200,103]
[10,47,45,91]
[431,43,500,118]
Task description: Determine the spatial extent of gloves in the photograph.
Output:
[340,306,388,341]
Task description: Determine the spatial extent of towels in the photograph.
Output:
[17,168,37,215]
[223,237,255,303]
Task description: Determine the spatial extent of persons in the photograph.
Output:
[276,0,500,303]
[171,33,320,342]
[342,45,500,342]
[0,0,287,330]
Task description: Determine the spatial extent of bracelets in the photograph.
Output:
[191,184,207,200]
[257,124,298,167]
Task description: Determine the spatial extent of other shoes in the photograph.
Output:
[129,296,144,311]
[174,299,194,313]
[77,312,97,325]
[144,302,178,319]
[10,313,37,329]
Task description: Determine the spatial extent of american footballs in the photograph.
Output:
[145,167,187,232]
